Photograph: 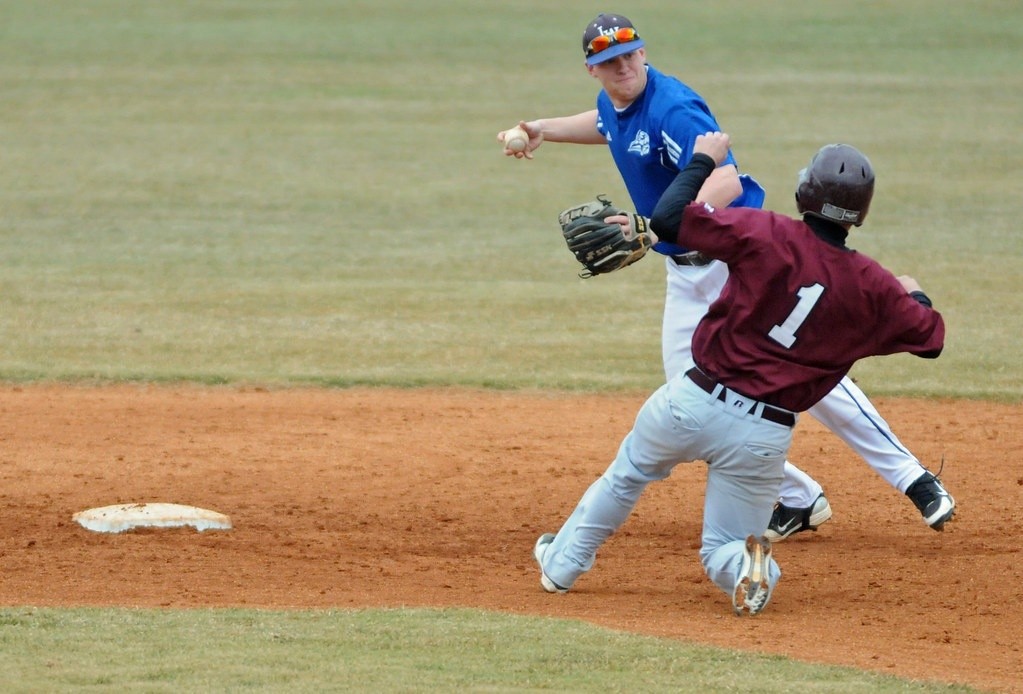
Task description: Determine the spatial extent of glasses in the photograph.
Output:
[586,27,640,59]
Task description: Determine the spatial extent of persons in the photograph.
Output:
[532,130,945,616]
[497,13,956,542]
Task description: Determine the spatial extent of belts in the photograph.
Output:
[670,251,713,266]
[686,367,795,426]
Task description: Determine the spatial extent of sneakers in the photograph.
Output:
[732,533,772,616]
[535,533,568,593]
[764,491,832,542]
[905,454,957,532]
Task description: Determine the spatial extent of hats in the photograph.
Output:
[582,13,643,65]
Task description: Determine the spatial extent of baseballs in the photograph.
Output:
[503,126,530,154]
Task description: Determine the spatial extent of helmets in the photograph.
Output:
[795,143,875,227]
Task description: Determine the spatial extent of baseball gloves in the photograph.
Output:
[558,200,658,277]
